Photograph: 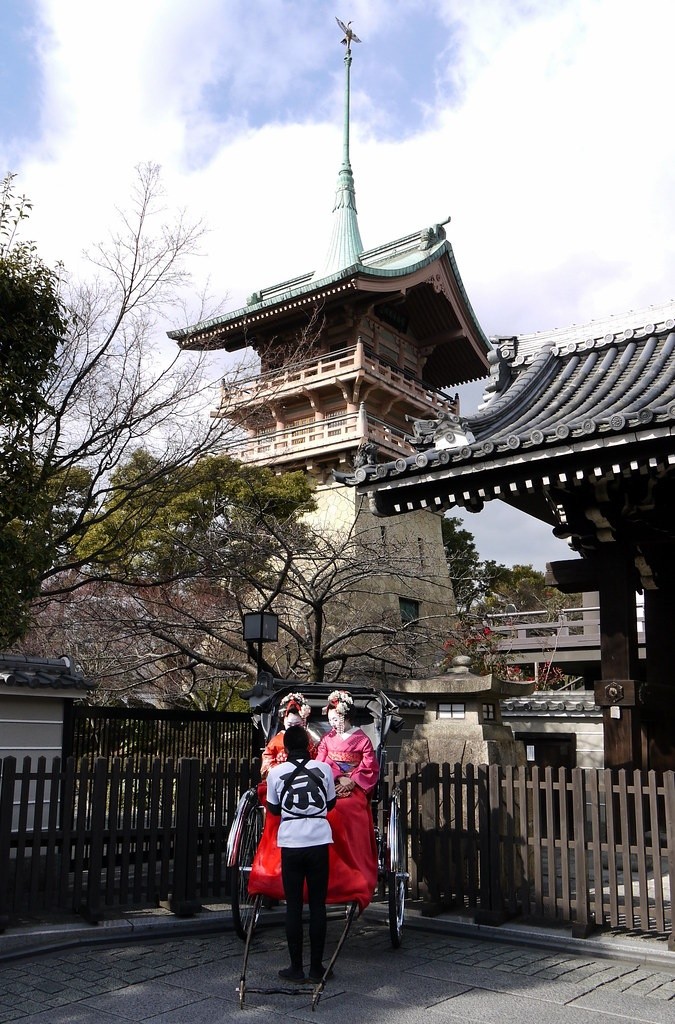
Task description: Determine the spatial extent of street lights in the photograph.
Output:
[242,611,279,678]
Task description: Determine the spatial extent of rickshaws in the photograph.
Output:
[224,670,410,1012]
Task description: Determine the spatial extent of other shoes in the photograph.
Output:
[278,966,336,985]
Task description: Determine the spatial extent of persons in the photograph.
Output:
[247,692,321,895]
[265,725,338,982]
[313,691,383,909]
